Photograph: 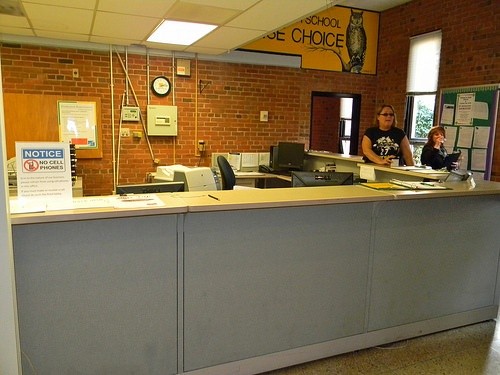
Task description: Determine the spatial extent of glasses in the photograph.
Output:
[379,113,394,116]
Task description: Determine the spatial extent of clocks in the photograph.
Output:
[152,77,171,96]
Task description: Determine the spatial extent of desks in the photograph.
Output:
[12,148,500,375]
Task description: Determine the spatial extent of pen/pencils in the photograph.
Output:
[208,194,220,201]
[420,182,435,186]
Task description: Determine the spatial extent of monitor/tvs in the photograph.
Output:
[289,170,354,186]
[268,140,305,173]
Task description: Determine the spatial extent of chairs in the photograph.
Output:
[218,155,236,190]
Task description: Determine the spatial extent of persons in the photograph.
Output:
[420,126,461,170]
[362,104,414,167]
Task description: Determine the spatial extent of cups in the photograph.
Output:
[147,176,154,183]
